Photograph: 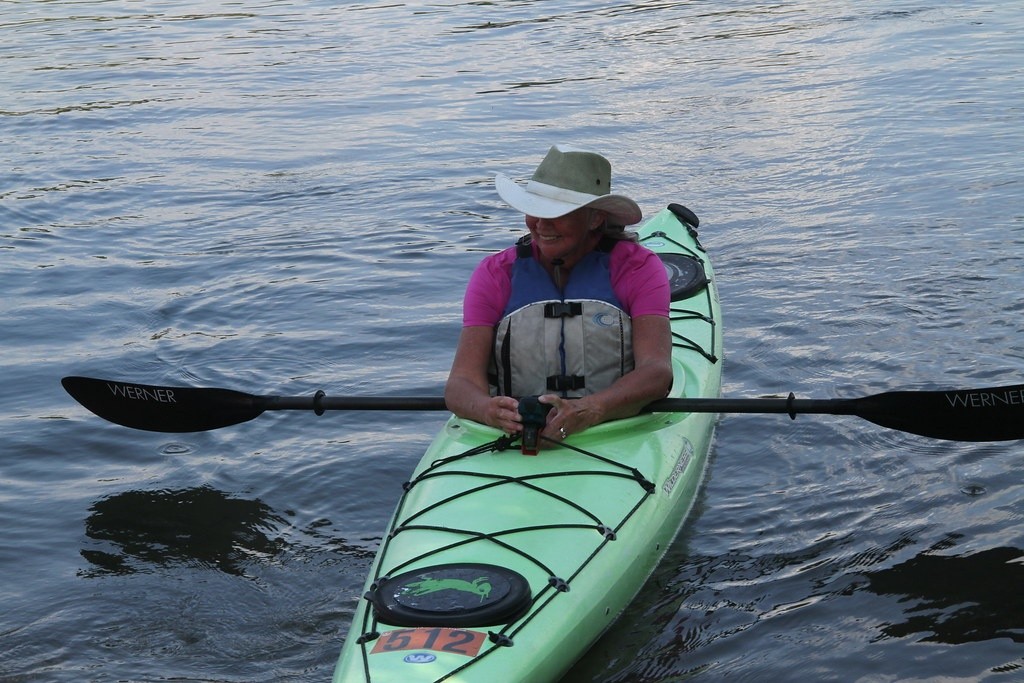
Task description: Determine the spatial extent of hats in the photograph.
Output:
[496,144,641,226]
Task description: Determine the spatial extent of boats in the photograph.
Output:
[333,203,725,682]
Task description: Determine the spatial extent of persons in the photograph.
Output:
[445,146,674,445]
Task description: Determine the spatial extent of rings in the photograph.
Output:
[560,428,567,438]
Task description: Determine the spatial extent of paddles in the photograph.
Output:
[60,373,1024,443]
